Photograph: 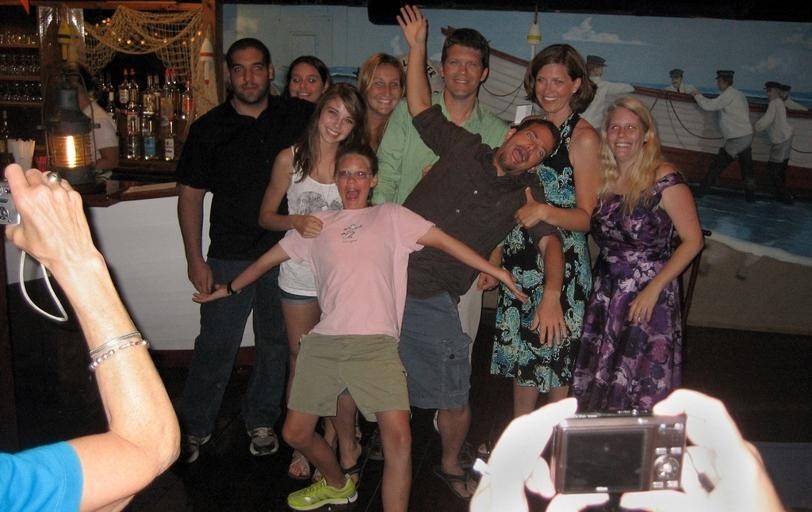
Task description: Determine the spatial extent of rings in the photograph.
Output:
[47,171,63,186]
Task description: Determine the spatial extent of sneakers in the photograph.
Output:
[287,474,358,510]
[245,423,280,456]
[178,430,213,463]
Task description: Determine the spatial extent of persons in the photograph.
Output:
[455,389,787,512]
[174,3,707,512]
[686,69,757,205]
[1,163,182,512]
[63,62,121,171]
[751,79,794,204]
[779,85,807,111]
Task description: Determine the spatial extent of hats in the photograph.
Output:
[715,69,736,80]
[763,81,782,90]
[669,69,684,78]
[781,85,792,91]
[586,55,606,66]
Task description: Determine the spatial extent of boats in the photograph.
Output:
[428,25,811,202]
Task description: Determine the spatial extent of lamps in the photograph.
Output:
[37,70,100,185]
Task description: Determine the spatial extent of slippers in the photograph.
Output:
[432,463,478,501]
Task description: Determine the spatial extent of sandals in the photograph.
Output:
[288,451,311,479]
[340,447,362,488]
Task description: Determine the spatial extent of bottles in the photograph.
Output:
[1,22,43,102]
[87,66,190,162]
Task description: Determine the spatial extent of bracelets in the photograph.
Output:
[88,339,151,373]
[89,330,142,356]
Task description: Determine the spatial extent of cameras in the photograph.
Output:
[548,409,686,494]
[0,182,21,224]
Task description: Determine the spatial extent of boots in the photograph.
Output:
[741,169,756,203]
[693,159,725,198]
[769,175,795,205]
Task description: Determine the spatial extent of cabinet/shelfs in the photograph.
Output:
[0,43,41,107]
